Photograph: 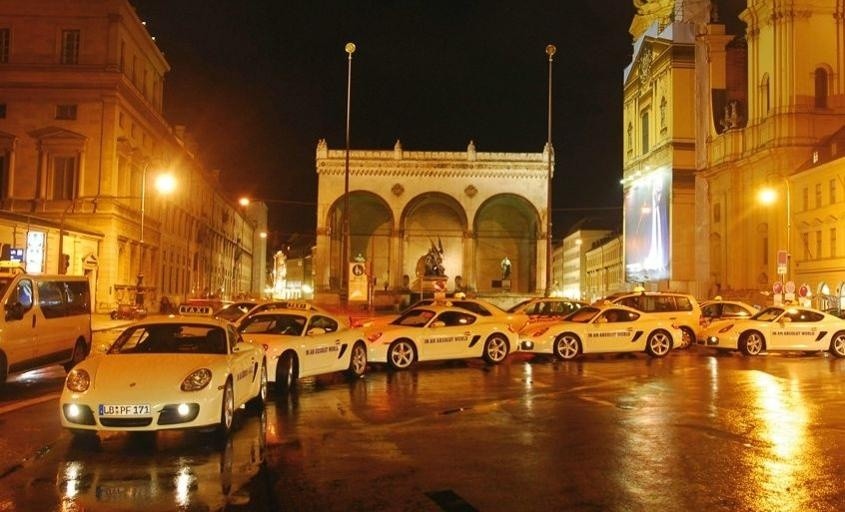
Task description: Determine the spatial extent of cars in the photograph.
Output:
[357,291,532,334]
[696,296,793,346]
[505,294,590,342]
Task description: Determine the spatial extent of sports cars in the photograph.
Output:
[516,298,684,363]
[700,300,845,363]
[213,297,333,332]
[350,303,522,371]
[57,299,269,445]
[239,297,370,399]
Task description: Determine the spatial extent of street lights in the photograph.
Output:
[753,170,792,282]
[130,155,181,322]
[337,42,358,310]
[543,42,559,298]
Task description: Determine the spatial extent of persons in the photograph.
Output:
[350,251,366,263]
[550,142,555,163]
[543,143,550,162]
[320,138,328,158]
[500,255,513,280]
[466,140,477,162]
[314,137,321,159]
[634,169,671,278]
[394,139,403,161]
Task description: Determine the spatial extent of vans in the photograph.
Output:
[604,288,708,351]
[1,271,92,384]
